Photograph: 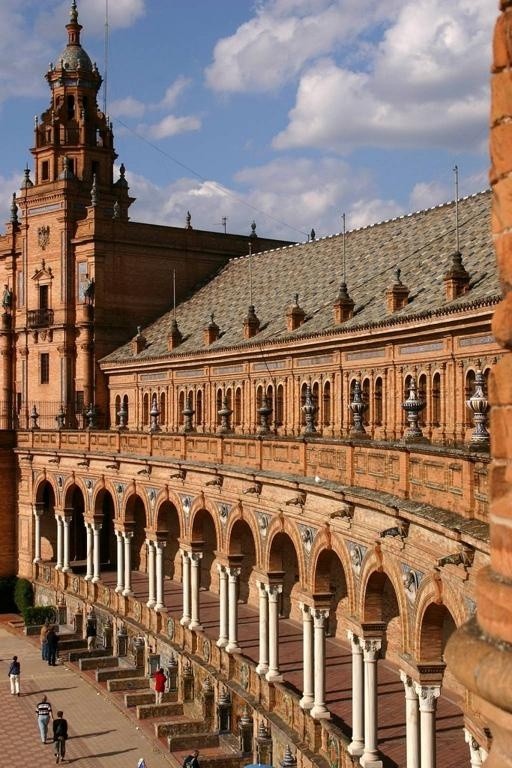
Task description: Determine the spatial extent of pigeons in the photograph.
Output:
[314,475,326,484]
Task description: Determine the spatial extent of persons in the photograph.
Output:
[82,274,92,305]
[36,694,54,744]
[9,656,20,697]
[153,668,167,704]
[52,710,68,764]
[182,749,200,768]
[41,622,60,666]
[1,284,10,314]
[85,623,97,650]
